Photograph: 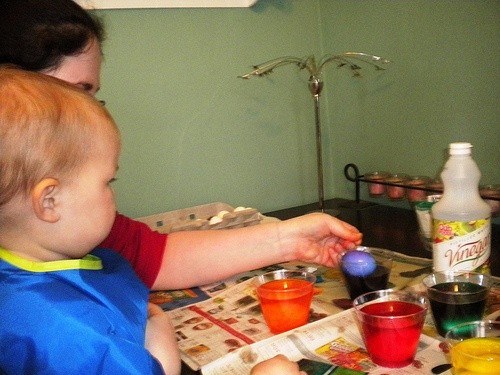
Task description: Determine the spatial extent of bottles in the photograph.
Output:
[431,142,492,286]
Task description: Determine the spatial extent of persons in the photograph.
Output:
[249,352,307,375]
[0,65,182,375]
[0,1,363,290]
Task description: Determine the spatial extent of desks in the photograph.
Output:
[262,198,500,277]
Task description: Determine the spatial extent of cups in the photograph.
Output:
[353,289,430,368]
[405,176,429,201]
[445,320,500,375]
[364,171,389,195]
[336,247,393,305]
[383,173,407,198]
[422,271,491,340]
[251,271,313,335]
[479,184,500,215]
[427,180,443,195]
